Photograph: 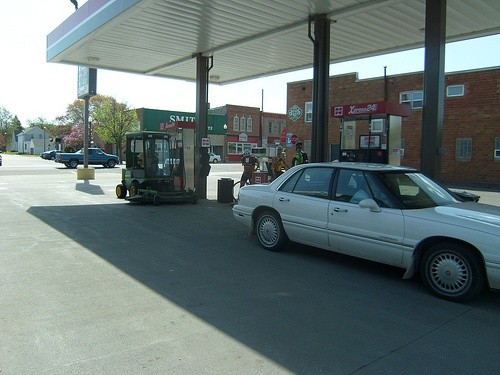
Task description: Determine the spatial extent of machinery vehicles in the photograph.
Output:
[115,130,199,206]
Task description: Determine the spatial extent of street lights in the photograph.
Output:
[223,124,228,163]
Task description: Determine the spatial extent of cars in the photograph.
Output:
[55,148,120,169]
[232,161,500,301]
[208,152,221,164]
[40,150,66,160]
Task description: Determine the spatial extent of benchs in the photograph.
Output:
[296,180,329,192]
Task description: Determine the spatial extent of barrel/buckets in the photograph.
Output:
[218,180,234,202]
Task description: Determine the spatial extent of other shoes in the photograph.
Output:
[235,199,238,201]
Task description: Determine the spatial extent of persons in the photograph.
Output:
[240,149,259,188]
[292,141,308,168]
[137,142,159,173]
[350,178,375,203]
[271,146,285,181]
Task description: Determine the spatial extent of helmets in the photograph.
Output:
[243,149,250,155]
[276,146,283,151]
[296,141,303,147]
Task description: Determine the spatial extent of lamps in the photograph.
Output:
[210,76,218,81]
[86,57,99,63]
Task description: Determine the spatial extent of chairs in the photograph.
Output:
[132,152,141,170]
